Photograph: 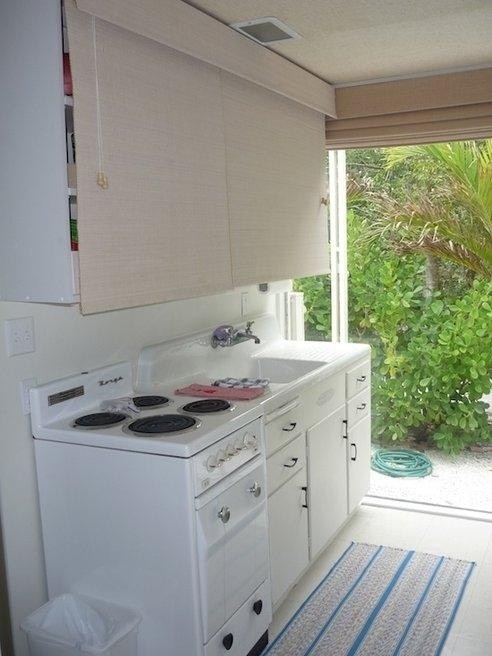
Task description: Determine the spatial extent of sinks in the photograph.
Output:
[214,357,327,384]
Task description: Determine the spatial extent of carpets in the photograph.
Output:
[262,542,475,656]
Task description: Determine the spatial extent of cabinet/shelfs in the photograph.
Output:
[1,1,340,318]
[179,412,269,652]
[261,347,373,610]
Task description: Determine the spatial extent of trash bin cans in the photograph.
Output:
[20,593,143,656]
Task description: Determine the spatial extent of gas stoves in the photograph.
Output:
[70,394,263,497]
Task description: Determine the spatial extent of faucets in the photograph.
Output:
[234,331,260,344]
